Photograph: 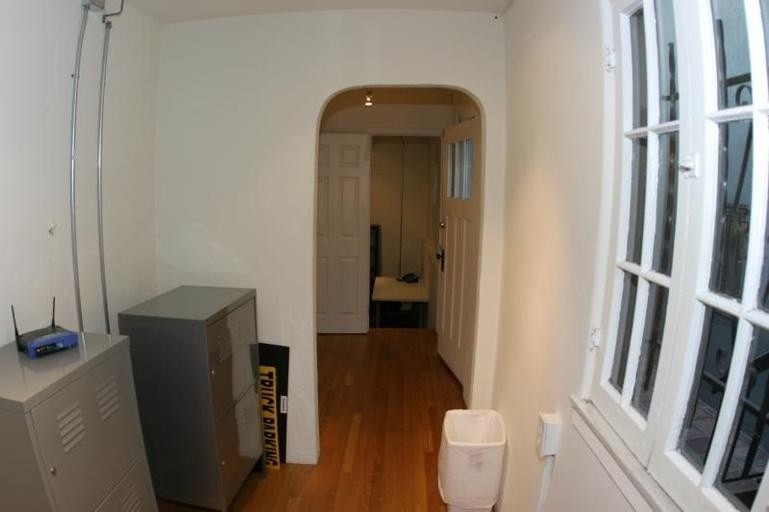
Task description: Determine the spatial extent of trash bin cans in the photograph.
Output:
[443,410,506,512]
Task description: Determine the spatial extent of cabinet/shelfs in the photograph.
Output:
[0,332,159,512]
[117,286,267,512]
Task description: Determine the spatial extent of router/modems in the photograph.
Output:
[10,296,79,358]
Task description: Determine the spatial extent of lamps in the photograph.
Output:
[359,88,377,111]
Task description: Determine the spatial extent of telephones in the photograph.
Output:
[403,272,418,283]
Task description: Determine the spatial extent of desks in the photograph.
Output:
[372,276,429,329]
